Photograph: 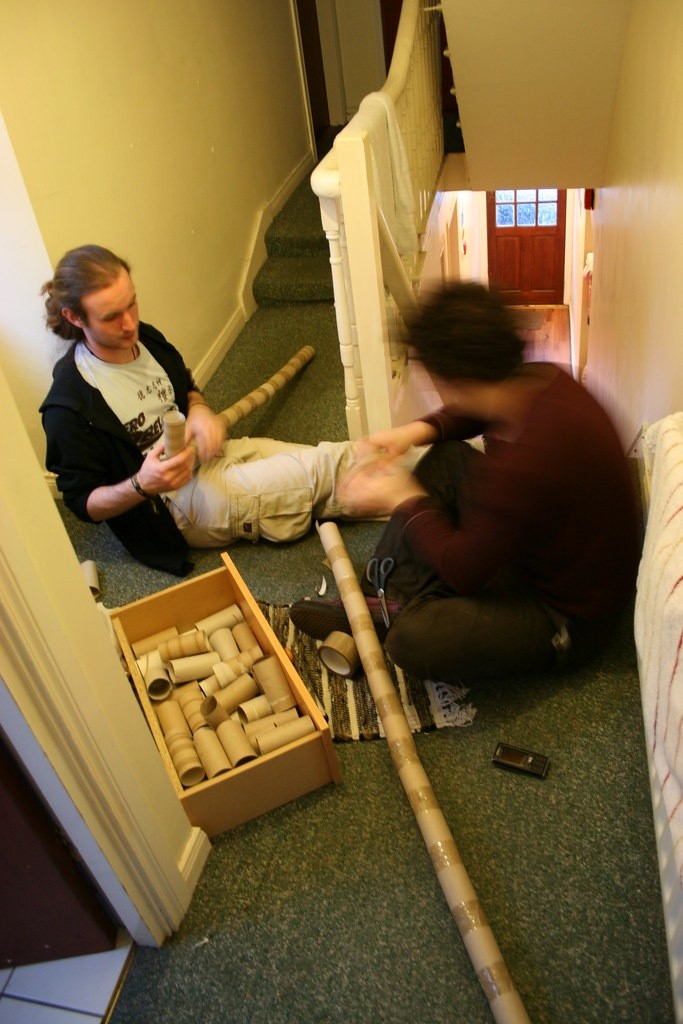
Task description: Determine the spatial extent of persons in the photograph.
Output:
[39,245,417,578]
[286,277,637,698]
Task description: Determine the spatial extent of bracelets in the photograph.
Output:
[131,474,154,499]
[189,401,213,410]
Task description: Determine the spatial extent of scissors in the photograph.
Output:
[366,558,394,630]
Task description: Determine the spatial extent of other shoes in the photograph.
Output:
[290,593,406,641]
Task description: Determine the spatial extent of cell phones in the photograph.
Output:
[491,740,550,779]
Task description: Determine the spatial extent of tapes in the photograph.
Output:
[317,632,361,677]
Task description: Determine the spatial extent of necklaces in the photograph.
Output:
[83,340,136,362]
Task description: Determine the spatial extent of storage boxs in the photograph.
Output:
[110,552,343,837]
